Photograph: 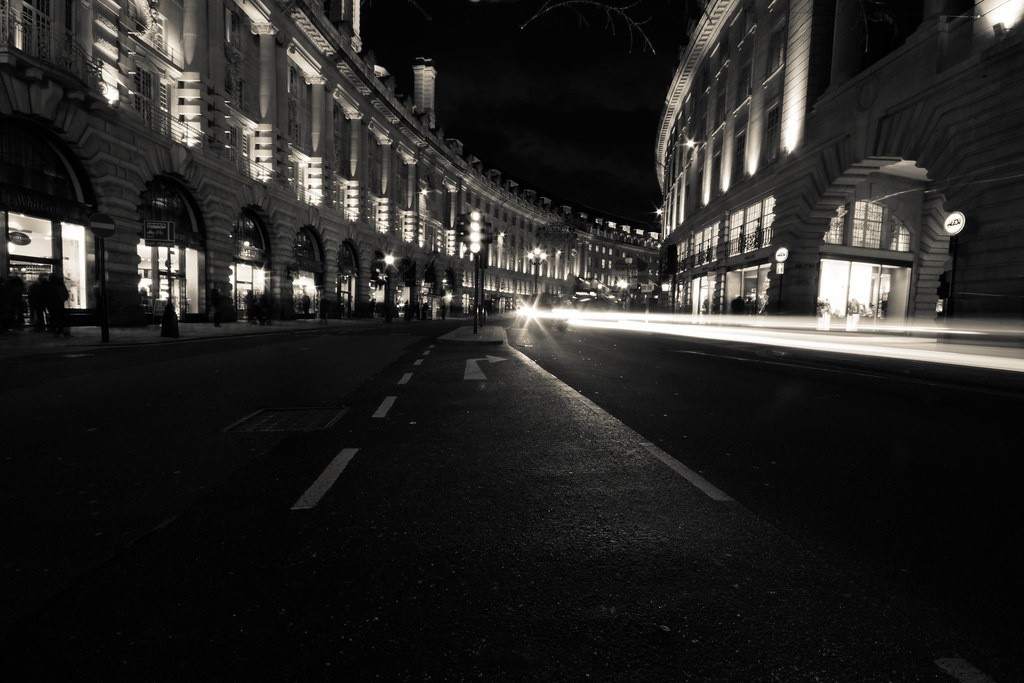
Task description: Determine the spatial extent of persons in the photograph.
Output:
[211,289,223,327]
[6,271,69,338]
[302,296,310,320]
[405,301,429,321]
[441,303,446,320]
[369,300,376,318]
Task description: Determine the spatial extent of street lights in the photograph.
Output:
[382,253,396,313]
[528,248,547,302]
[623,255,636,313]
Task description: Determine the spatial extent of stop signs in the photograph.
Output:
[89,214,117,238]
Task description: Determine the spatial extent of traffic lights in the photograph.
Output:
[455,209,493,257]
